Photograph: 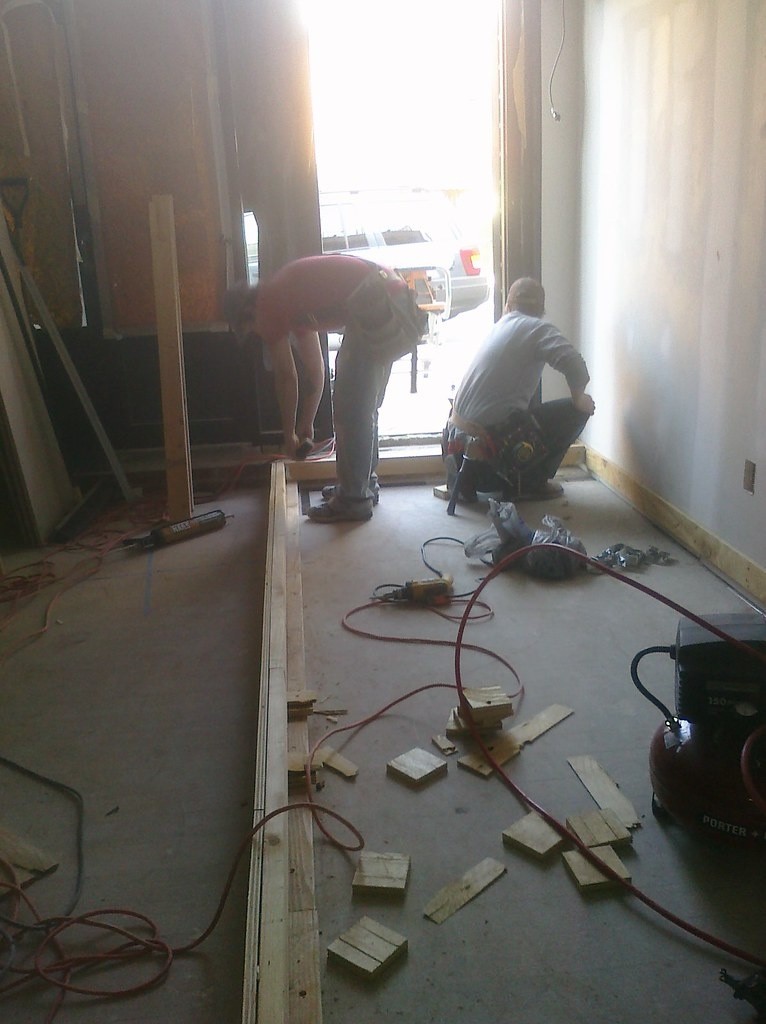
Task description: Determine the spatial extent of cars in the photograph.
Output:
[241,193,491,324]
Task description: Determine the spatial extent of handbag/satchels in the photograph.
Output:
[353,297,421,366]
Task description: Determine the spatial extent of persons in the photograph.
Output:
[442,278,596,498]
[218,254,427,523]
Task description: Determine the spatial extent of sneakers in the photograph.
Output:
[503,479,566,502]
[306,485,381,523]
[444,452,479,503]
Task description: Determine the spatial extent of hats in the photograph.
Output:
[220,281,255,352]
[508,276,545,304]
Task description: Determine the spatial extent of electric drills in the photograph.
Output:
[370,576,451,609]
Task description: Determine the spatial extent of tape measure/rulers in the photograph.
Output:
[515,441,534,463]
